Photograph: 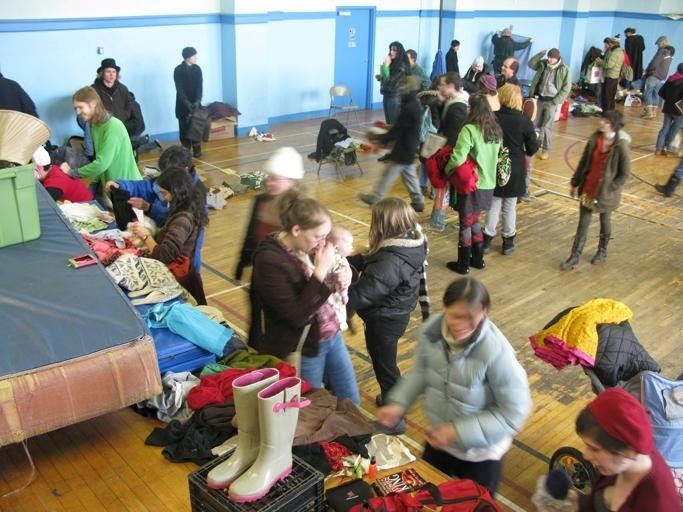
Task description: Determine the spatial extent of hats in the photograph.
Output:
[547,48,559,59]
[182,48,196,59]
[612,34,620,46]
[472,56,484,72]
[501,29,511,37]
[480,75,496,90]
[587,387,654,454]
[97,59,120,73]
[265,148,305,180]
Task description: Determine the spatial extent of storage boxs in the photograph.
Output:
[0,159,41,248]
[207,115,236,141]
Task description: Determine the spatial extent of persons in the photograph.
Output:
[61,57,146,209]
[375,276,530,494]
[105,145,209,305]
[528,48,571,159]
[569,387,683,512]
[359,27,543,274]
[642,36,675,117]
[561,110,631,271]
[656,63,683,196]
[347,197,430,434]
[237,145,360,408]
[174,47,203,157]
[625,27,645,81]
[597,34,630,111]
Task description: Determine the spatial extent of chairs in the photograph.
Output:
[317,119,363,181]
[328,86,358,125]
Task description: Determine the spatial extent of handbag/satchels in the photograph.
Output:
[585,65,601,84]
[620,64,633,82]
[496,145,511,186]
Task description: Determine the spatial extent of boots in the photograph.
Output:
[561,236,586,270]
[655,175,680,197]
[207,368,279,489]
[592,233,609,265]
[411,186,533,273]
[640,106,657,118]
[228,376,311,502]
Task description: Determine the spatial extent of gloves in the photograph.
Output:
[185,99,200,112]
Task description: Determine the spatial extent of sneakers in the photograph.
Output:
[358,192,376,204]
